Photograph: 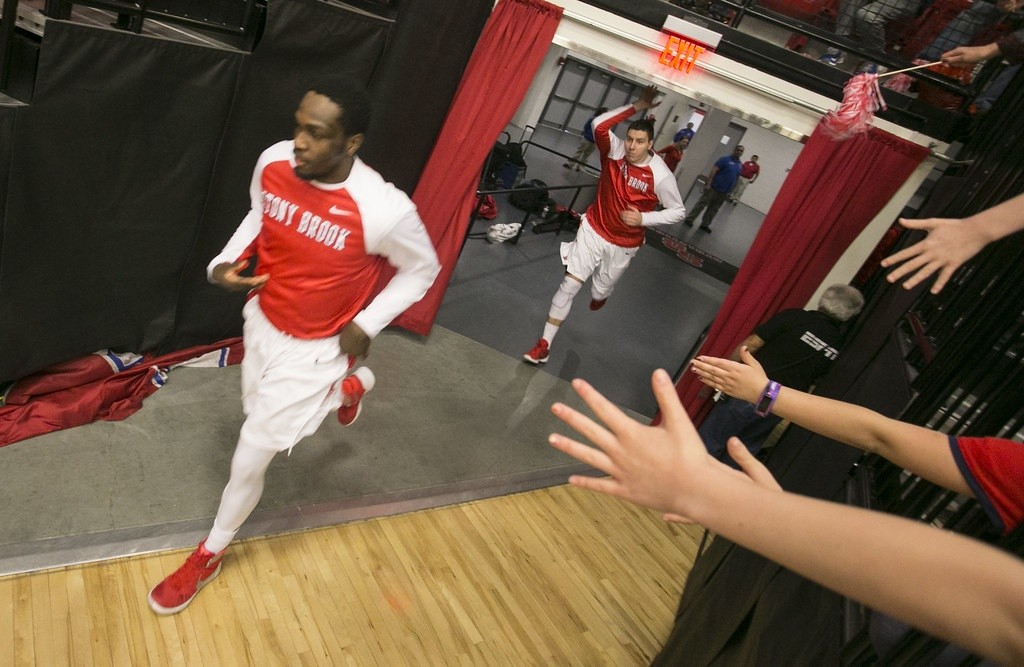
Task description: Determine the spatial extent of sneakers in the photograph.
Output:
[523,338,550,363]
[589,297,607,311]
[338,366,376,426]
[149,538,229,614]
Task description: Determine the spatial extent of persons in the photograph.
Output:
[523,85,687,364]
[684,145,744,234]
[549,0,1023,667]
[729,155,760,206]
[699,285,864,471]
[148,66,441,616]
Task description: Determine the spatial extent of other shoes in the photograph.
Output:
[685,219,693,227]
[700,225,712,234]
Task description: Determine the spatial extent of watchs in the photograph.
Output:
[753,381,781,417]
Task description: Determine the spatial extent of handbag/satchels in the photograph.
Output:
[487,222,522,244]
[508,179,580,234]
[474,194,497,220]
